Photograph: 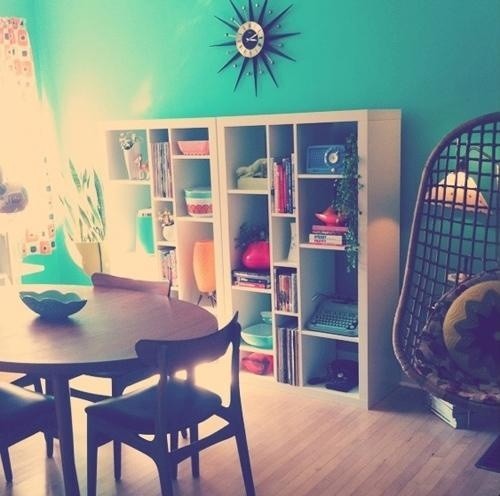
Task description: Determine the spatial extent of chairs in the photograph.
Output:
[391,103,498,429]
[0,271,258,496]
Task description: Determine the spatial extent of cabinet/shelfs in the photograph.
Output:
[218,109,401,410]
[102,118,221,319]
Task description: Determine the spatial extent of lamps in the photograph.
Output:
[421,140,499,215]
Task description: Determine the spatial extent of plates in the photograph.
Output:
[18,289,89,319]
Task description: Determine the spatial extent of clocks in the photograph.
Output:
[237,20,264,58]
